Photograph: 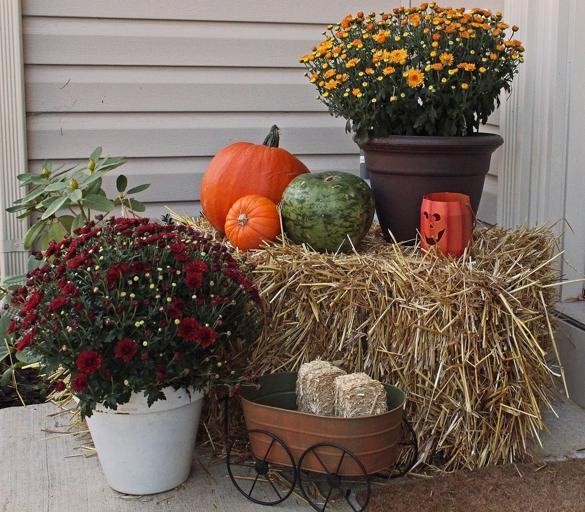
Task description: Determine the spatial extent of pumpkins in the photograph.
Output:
[223,194,281,250]
[282,172,376,253]
[199,124,311,235]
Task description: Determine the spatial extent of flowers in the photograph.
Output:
[4,214,264,423]
[298,1,526,145]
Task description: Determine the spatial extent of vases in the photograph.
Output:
[352,134,504,247]
[73,382,211,495]
